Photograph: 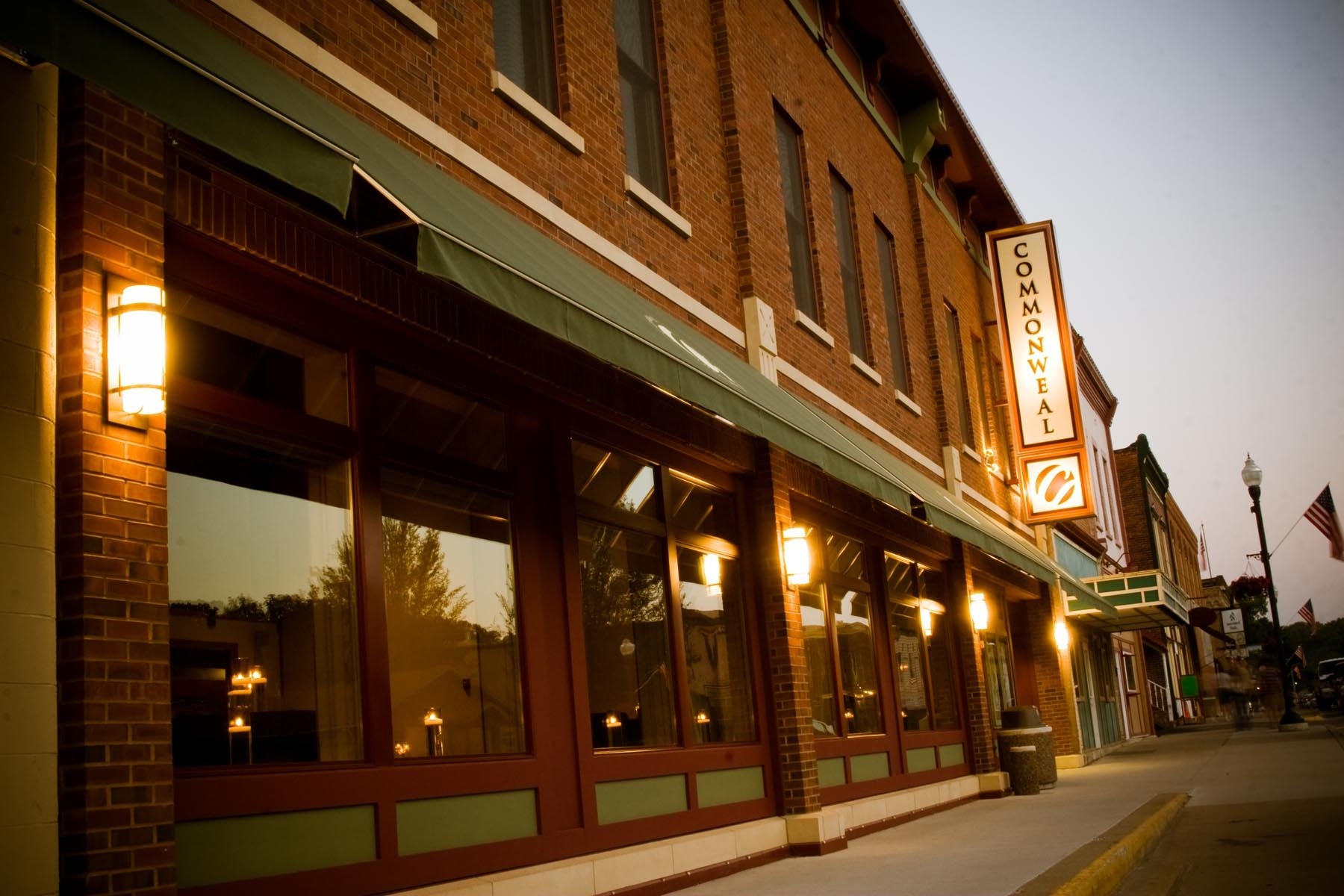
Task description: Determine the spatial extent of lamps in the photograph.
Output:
[227,658,267,765]
[103,272,167,432]
[606,711,623,747]
[1055,620,1069,652]
[970,592,988,633]
[781,521,810,591]
[920,608,932,635]
[423,707,444,757]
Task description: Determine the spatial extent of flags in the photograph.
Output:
[1200,534,1206,570]
[1294,666,1300,676]
[1297,599,1316,635]
[1295,644,1307,668]
[1303,484,1344,561]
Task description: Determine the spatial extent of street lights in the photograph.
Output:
[1242,453,1308,731]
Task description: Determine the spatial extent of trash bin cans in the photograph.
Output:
[1009,747,1042,797]
[996,705,1059,791]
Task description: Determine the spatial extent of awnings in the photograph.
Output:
[0,0,1120,621]
[1198,626,1237,669]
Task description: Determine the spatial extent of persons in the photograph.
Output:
[1256,659,1283,727]
[1217,659,1254,722]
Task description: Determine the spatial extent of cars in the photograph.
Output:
[1314,657,1344,710]
